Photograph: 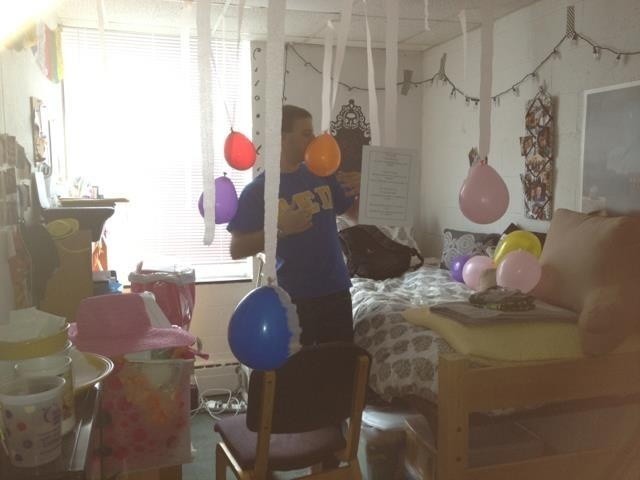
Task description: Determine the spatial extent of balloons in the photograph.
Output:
[304,129,340,177]
[198,127,256,224]
[228,285,303,372]
[450,161,542,294]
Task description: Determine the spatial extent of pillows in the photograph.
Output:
[438,223,546,272]
[530,207,640,354]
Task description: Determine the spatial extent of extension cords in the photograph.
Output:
[208,400,245,413]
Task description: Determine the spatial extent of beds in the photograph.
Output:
[337,226,640,479]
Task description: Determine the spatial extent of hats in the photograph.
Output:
[66,291,197,355]
[45,218,79,239]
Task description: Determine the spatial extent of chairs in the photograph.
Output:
[214,339,372,476]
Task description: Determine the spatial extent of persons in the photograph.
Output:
[227,105,362,474]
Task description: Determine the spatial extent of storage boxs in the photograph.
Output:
[100,354,195,476]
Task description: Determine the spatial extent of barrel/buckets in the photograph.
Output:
[0,322,76,468]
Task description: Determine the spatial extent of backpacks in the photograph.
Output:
[338,225,424,281]
